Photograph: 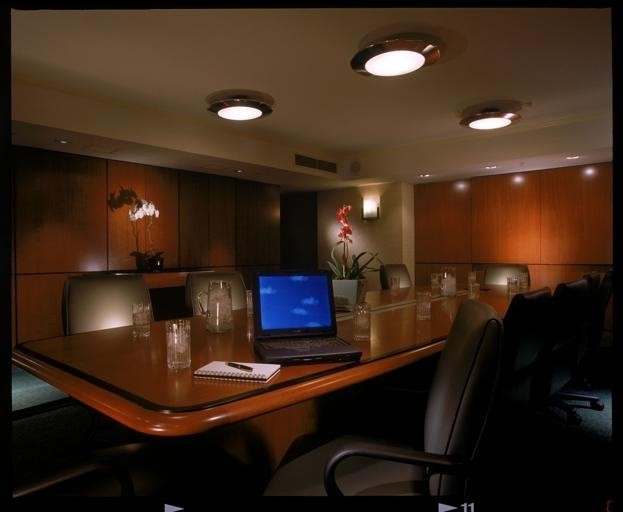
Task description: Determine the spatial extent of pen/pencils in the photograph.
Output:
[226,362,254,372]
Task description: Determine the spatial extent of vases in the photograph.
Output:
[322,205,384,280]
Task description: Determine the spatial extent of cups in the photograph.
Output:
[432,272,442,290]
[519,272,528,290]
[351,303,372,341]
[390,276,401,296]
[416,287,432,321]
[246,289,254,319]
[133,302,151,338]
[469,283,480,300]
[507,274,518,295]
[165,320,193,369]
[466,271,477,289]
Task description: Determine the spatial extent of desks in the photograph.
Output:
[12,279,557,497]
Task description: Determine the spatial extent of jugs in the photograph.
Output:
[196,280,235,333]
[441,269,456,297]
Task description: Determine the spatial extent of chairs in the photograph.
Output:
[186,271,248,315]
[61,272,156,497]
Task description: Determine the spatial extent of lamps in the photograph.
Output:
[351,38,441,79]
[459,109,522,130]
[361,199,380,222]
[207,96,273,121]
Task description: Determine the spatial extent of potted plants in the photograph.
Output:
[144,250,167,272]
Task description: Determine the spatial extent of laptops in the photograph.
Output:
[253,270,362,365]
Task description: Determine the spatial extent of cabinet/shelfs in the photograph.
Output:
[12,147,280,349]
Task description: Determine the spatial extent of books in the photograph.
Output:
[193,360,281,384]
